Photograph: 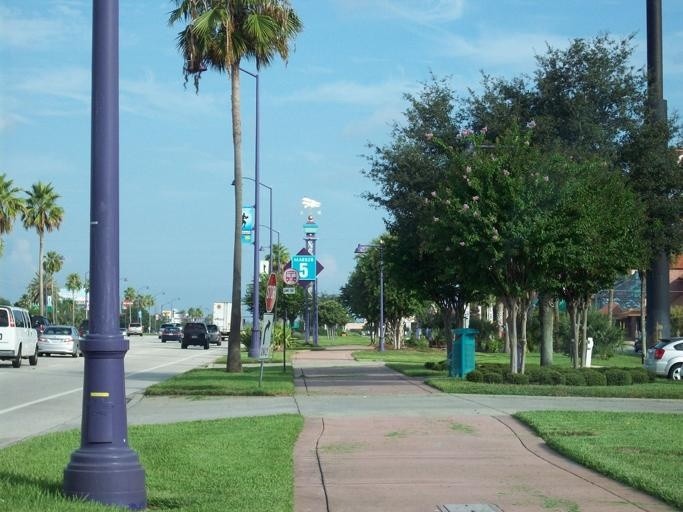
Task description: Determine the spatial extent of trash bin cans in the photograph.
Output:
[450,329,479,379]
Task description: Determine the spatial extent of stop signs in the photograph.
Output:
[266,274,276,312]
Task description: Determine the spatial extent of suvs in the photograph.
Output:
[31,314,53,338]
[158,322,174,339]
[182,322,209,350]
[127,322,145,337]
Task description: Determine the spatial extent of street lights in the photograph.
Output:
[119,277,127,283]
[184,49,260,358]
[228,174,274,275]
[127,286,182,333]
[355,239,387,353]
[83,269,89,320]
[259,243,271,252]
[252,223,282,325]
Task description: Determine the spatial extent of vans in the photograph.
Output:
[1,305,40,369]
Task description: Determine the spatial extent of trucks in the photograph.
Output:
[210,301,246,343]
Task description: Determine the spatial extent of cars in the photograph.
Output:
[205,324,222,347]
[38,325,84,359]
[634,335,642,353]
[78,319,90,339]
[119,327,128,336]
[643,337,683,380]
[162,327,182,343]
